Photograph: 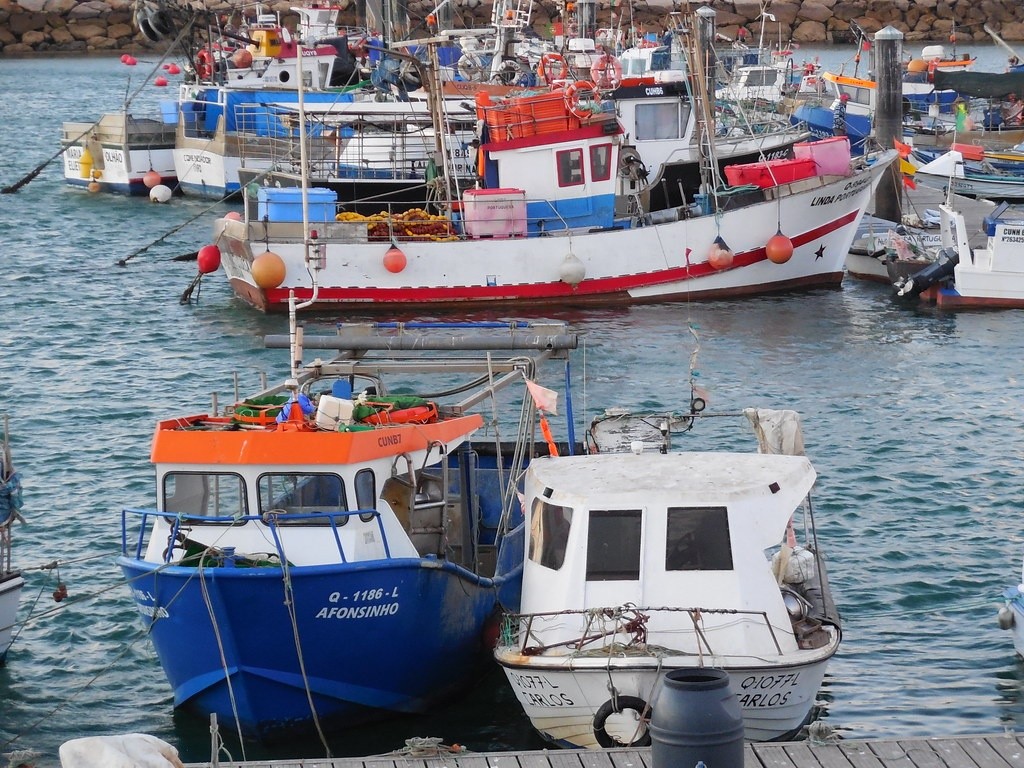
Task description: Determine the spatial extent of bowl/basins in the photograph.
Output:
[782,591,804,619]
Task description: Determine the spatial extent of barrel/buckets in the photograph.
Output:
[650,668,745,768]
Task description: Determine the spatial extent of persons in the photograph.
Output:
[832,93,851,137]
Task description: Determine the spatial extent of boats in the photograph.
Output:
[493,244,846,748]
[120,316,577,743]
[51,0,1024,323]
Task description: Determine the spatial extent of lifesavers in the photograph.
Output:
[195,50,216,79]
[495,60,522,87]
[564,79,601,118]
[590,54,622,88]
[537,53,568,84]
[458,53,483,82]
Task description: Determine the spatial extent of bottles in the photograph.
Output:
[316,395,354,431]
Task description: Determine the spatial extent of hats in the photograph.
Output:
[840,94,851,100]
